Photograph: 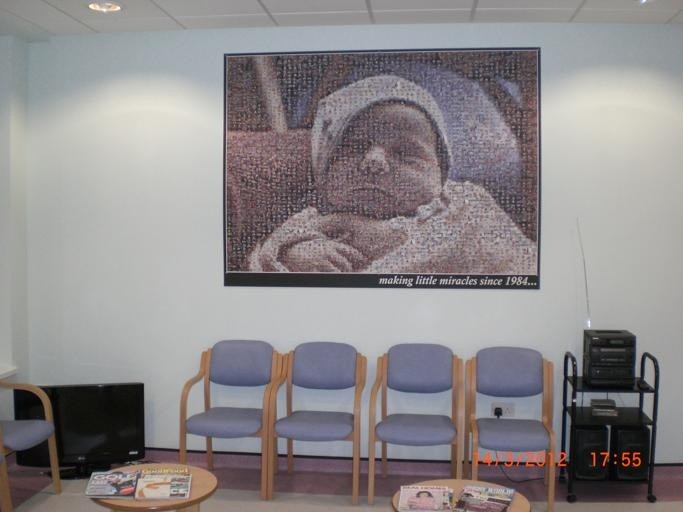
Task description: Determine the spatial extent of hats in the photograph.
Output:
[309,74,450,189]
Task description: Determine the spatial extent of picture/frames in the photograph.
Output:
[223,47,541,290]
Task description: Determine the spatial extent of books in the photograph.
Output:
[83,468,139,500]
[451,483,515,512]
[447,488,454,504]
[392,481,452,511]
[134,472,192,500]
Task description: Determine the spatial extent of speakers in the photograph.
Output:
[570,425,609,481]
[609,425,650,481]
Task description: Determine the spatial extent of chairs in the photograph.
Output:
[267,342,367,507]
[0,379,62,512]
[366,343,465,503]
[466,346,556,510]
[182,340,283,501]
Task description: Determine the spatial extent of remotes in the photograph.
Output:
[637,380,649,390]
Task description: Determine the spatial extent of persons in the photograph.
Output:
[241,72,539,277]
[407,490,440,511]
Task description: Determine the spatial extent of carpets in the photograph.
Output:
[11,479,681,512]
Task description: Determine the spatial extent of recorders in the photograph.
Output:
[584,329,636,388]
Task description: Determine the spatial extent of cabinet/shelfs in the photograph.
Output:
[560,351,660,504]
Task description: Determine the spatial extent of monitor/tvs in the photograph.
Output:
[13,381,145,481]
